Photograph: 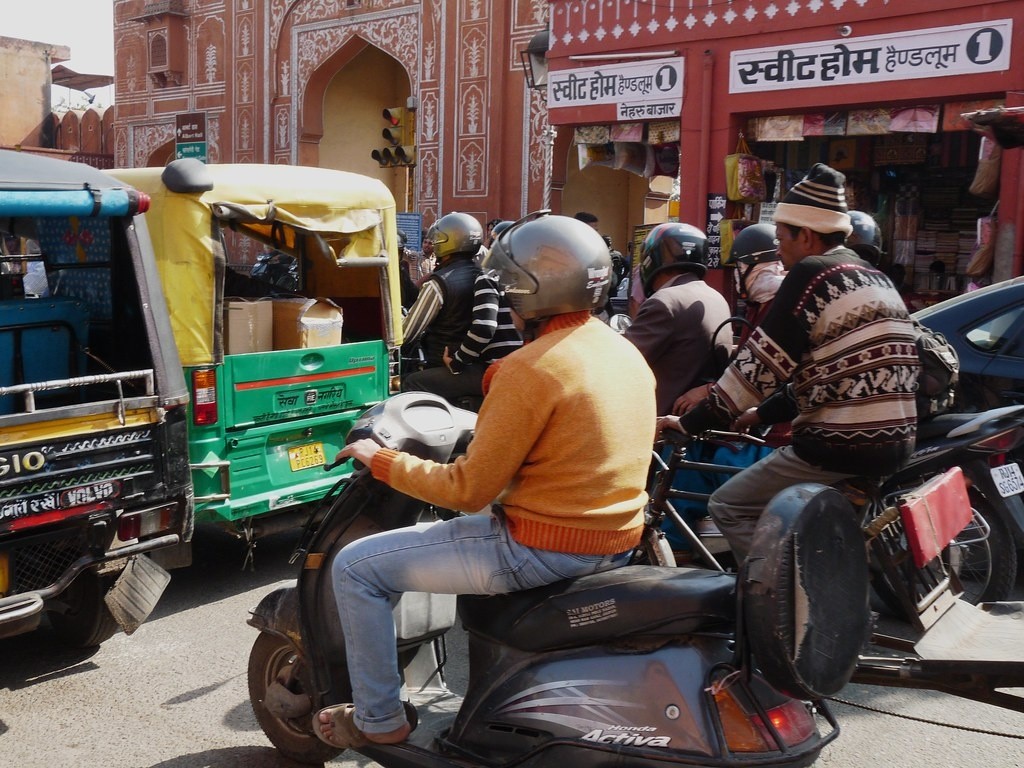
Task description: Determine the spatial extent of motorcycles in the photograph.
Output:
[609,314,1024,606]
[99,163,404,527]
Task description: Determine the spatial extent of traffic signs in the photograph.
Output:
[175,110,208,164]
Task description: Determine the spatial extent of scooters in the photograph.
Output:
[246,391,873,768]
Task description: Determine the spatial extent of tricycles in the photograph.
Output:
[627,427,1024,713]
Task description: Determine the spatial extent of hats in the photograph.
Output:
[771,163,853,239]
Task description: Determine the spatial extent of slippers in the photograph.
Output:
[312,701,418,748]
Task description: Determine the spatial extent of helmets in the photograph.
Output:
[725,223,782,264]
[481,209,612,321]
[426,213,483,258]
[844,211,882,258]
[639,223,709,298]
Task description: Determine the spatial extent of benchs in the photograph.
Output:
[1,296,91,411]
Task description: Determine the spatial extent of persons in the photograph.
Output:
[660,165,920,570]
[309,203,660,753]
[622,219,734,422]
[392,214,523,408]
[657,207,886,567]
[575,210,635,326]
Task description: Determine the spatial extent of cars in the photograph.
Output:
[908,275,1024,477]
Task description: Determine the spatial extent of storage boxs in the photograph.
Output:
[221,295,344,355]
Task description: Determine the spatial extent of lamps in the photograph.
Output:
[520,21,550,104]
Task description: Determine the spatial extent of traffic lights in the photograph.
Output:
[371,106,417,168]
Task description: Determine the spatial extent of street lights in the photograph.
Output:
[519,20,552,209]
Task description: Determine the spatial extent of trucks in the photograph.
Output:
[0,148,195,649]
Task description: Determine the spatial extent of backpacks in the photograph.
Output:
[912,318,961,420]
